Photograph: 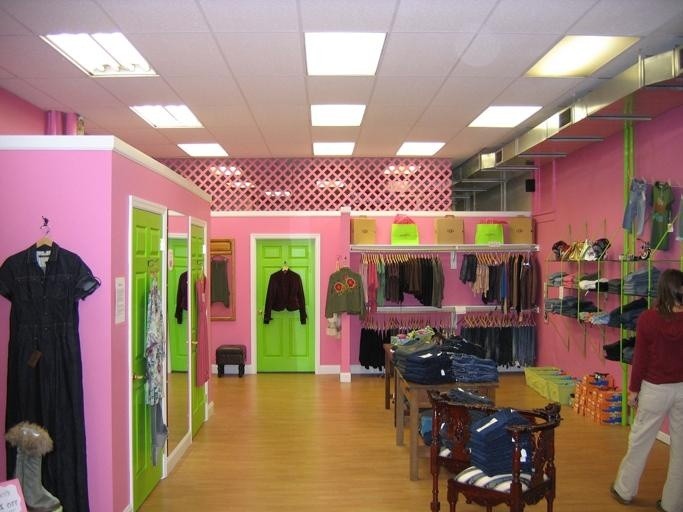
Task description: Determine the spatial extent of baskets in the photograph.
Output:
[435,215,464,244]
[351,215,377,245]
[510,215,535,243]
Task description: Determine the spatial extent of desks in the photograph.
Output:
[391,365,499,480]
[384,341,399,427]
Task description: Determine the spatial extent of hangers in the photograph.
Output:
[359,247,536,266]
[278,260,290,273]
[208,253,229,262]
[363,314,537,331]
[34,221,56,252]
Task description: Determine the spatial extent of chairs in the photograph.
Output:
[427,400,562,511]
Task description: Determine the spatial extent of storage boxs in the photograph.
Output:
[524,366,626,427]
[350,216,535,247]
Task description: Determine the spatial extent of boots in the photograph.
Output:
[10,422,61,511]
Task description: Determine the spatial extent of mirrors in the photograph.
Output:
[165,207,190,461]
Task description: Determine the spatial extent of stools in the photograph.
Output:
[215,344,246,377]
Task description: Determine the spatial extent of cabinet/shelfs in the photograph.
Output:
[621,185,683,367]
[542,222,621,335]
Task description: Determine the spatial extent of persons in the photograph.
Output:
[609,269,683,512]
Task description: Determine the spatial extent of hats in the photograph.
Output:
[552,238,610,260]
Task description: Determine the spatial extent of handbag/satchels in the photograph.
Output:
[475,223,504,245]
[391,224,419,246]
